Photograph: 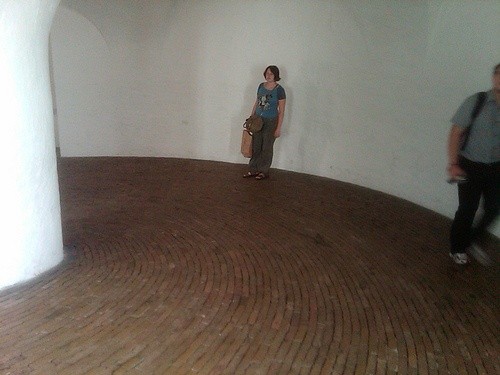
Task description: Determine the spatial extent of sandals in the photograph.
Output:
[254,172,268,180]
[243,170,258,178]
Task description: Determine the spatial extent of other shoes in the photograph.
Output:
[448,248,469,264]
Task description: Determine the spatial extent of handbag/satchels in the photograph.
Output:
[244,115,264,132]
[240,128,254,160]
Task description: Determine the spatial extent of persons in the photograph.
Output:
[243,65,286,180]
[448,64,500,265]
